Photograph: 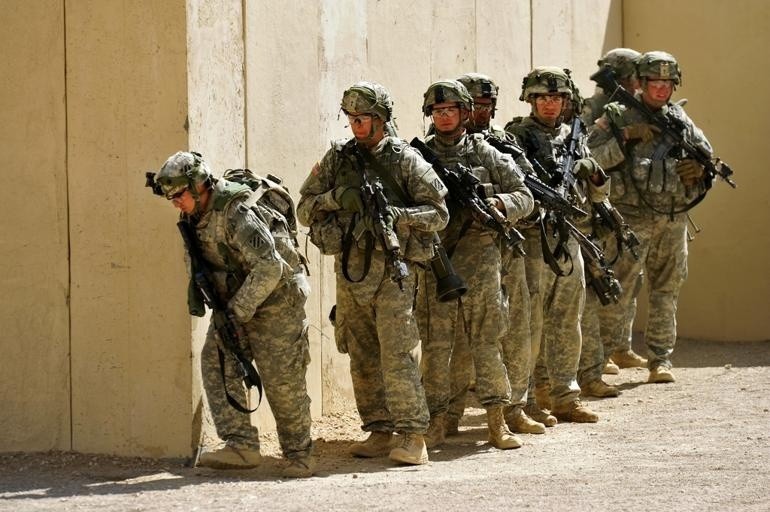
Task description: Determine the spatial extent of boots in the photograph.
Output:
[581,344,675,397]
[487,384,597,449]
[349,413,458,464]
[283,455,317,478]
[199,445,261,469]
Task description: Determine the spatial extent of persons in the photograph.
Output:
[422,49,715,448]
[297,83,450,466]
[144,151,314,478]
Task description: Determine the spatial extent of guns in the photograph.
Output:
[559,113,587,205]
[525,173,640,306]
[349,145,409,291]
[410,137,526,257]
[590,63,736,188]
[176,221,253,390]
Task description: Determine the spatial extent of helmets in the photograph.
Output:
[596,48,682,86]
[155,150,210,198]
[422,73,499,124]
[341,81,393,122]
[519,65,575,103]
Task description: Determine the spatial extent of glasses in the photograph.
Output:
[348,114,377,124]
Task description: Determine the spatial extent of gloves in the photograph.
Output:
[340,188,365,218]
[675,159,704,181]
[364,208,394,239]
[571,158,595,180]
[625,123,662,143]
